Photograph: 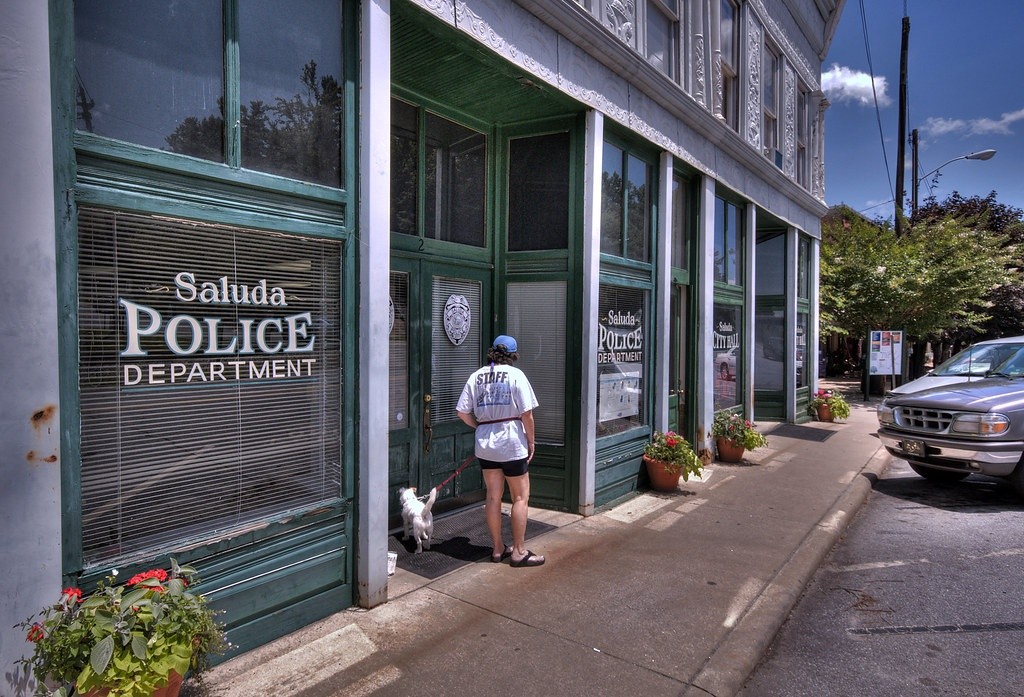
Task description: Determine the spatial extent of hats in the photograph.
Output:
[492,335,517,352]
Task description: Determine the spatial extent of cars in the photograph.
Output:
[715,346,803,385]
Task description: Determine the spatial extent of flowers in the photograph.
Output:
[643,431,704,481]
[808,389,849,419]
[713,410,768,451]
[13,558,240,697]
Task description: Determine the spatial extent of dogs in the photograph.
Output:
[399,487,437,554]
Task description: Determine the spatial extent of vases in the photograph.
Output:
[74,665,181,697]
[644,454,681,491]
[717,436,744,463]
[818,404,834,422]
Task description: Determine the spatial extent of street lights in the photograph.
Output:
[913,149,997,215]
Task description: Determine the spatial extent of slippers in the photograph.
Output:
[510,550,545,567]
[490,544,513,563]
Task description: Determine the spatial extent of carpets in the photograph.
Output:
[388,505,559,580]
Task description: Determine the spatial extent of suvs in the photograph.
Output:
[876,336,1024,490]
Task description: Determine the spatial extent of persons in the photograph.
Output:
[456,335,546,567]
